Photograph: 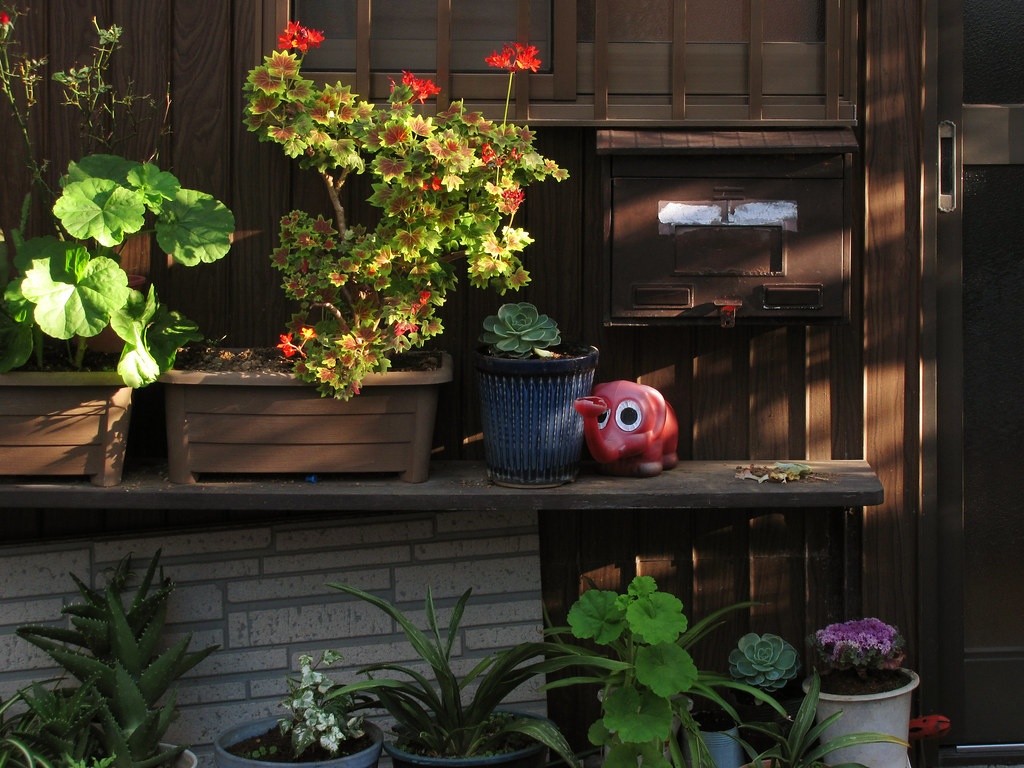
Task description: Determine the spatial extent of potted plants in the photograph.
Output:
[0,3,235,488]
[469,303,600,490]
[0,552,911,768]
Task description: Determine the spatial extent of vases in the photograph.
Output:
[801,668,920,767]
[162,345,454,486]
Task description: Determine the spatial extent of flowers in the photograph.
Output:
[239,22,572,404]
[805,617,906,688]
